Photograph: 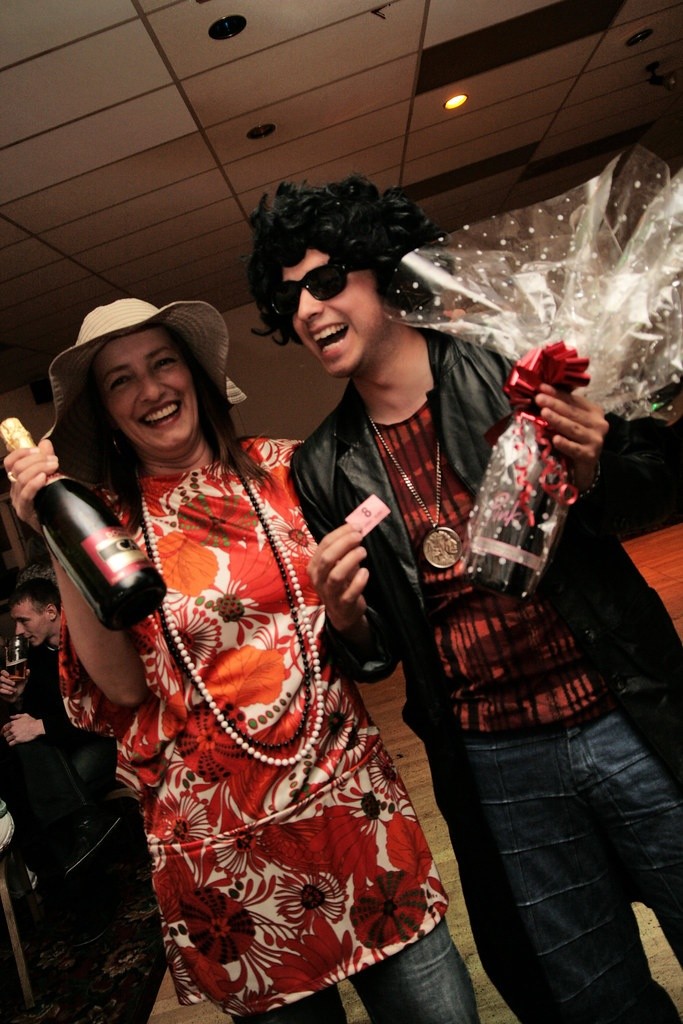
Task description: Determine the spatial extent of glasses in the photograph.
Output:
[271,260,371,317]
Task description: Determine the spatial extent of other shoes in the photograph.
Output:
[74,894,124,947]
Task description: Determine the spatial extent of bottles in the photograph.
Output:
[468,415,566,600]
[0,417,167,632]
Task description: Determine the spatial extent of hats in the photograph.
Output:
[39,298,247,486]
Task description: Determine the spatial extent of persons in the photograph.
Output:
[0,577,122,945]
[5,296,478,1024]
[244,173,683,1024]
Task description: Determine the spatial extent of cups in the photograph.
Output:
[4,637,29,681]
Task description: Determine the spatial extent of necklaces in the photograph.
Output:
[366,411,462,569]
[137,451,324,767]
